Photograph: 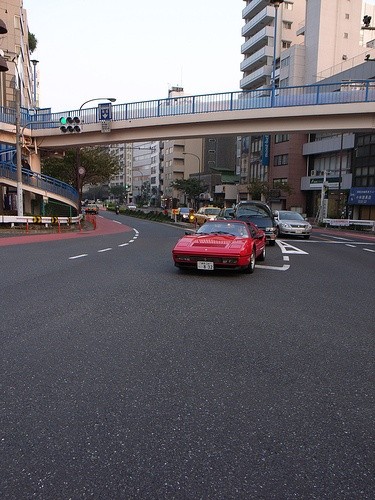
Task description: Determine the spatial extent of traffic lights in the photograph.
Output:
[59,116,80,125]
[59,126,80,133]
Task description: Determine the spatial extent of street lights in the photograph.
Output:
[182,152,200,210]
[30,60,39,109]
[79,98,116,116]
[269,0,284,88]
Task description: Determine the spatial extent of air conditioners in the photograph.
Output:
[310,169,318,176]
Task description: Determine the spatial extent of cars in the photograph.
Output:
[193,207,221,226]
[216,208,235,221]
[176,207,195,222]
[85,204,99,214]
[237,215,277,246]
[172,220,266,274]
[97,199,102,204]
[274,210,313,239]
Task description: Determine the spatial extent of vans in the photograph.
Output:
[126,204,137,210]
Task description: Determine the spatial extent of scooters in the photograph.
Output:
[115,206,119,215]
